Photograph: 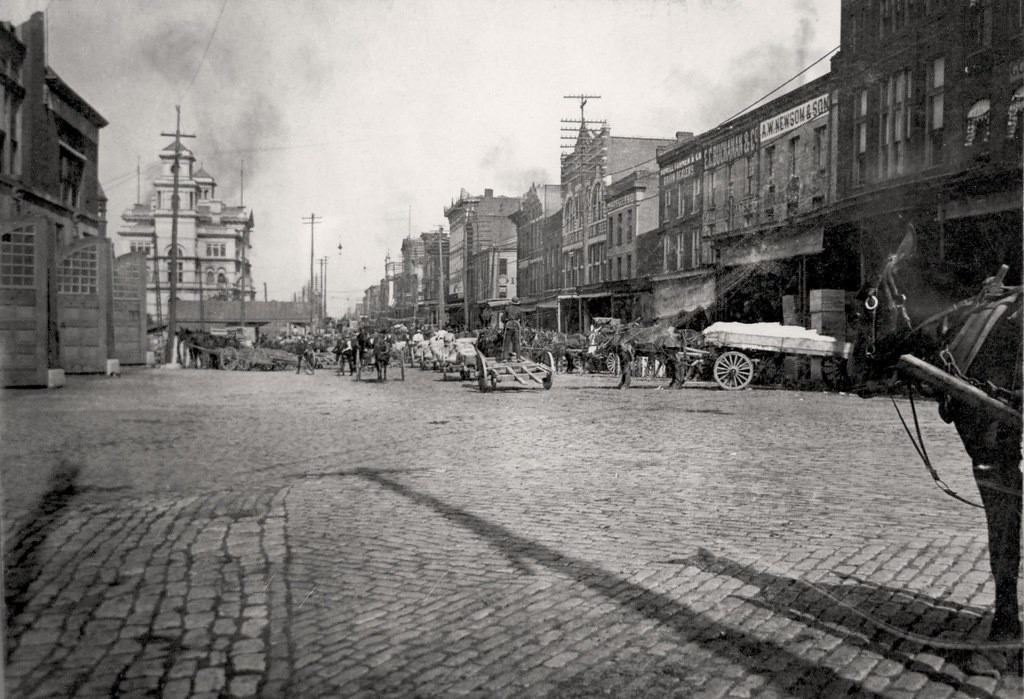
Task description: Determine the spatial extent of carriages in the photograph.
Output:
[582,309,853,390]
[144,317,657,382]
[472,322,554,393]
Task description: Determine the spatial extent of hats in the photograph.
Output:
[509,297,519,304]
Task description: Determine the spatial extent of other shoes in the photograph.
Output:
[502,359,508,362]
[517,359,522,362]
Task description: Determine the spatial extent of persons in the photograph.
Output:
[335,323,456,376]
[500,297,524,364]
[296,336,314,373]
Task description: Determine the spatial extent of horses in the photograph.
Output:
[519,316,688,393]
[178,326,244,370]
[371,330,391,382]
[846,222,1024,644]
